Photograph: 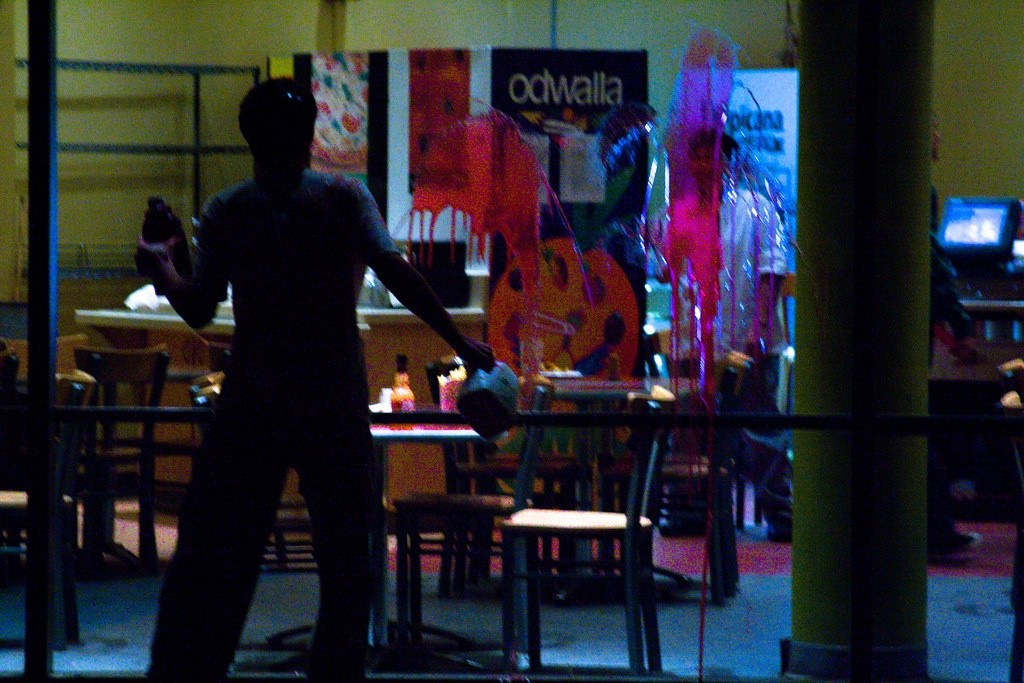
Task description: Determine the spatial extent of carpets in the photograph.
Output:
[380,517,1018,578]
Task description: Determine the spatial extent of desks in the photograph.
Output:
[75,305,488,511]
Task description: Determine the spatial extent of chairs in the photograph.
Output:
[0,339,740,676]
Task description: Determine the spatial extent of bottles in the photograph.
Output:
[391,373,414,412]
[142,197,190,296]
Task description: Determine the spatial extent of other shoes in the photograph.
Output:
[928,532,985,556]
[766,522,793,544]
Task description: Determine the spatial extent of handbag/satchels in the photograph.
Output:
[456,359,519,439]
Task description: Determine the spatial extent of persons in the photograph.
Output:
[134,78,494,683]
[927,117,991,565]
[658,128,791,539]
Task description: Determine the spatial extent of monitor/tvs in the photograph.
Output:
[936,195,1020,259]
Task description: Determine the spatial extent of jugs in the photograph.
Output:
[456,361,517,438]
